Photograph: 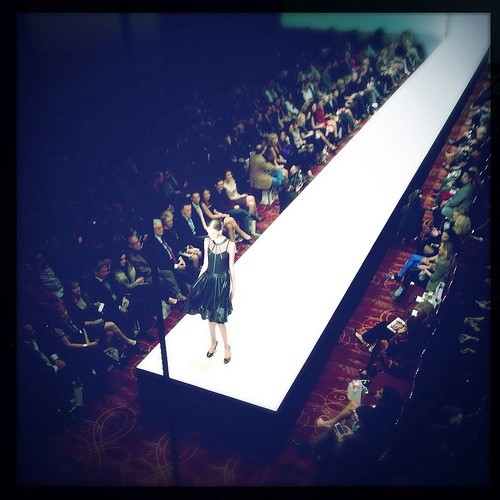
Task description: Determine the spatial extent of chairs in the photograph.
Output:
[364,141,490,464]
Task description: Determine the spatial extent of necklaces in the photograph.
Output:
[213,238,228,246]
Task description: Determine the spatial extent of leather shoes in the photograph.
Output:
[129,346,147,353]
[138,330,157,339]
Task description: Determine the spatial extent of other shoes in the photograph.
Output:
[238,233,251,240]
[427,111,474,211]
[176,293,186,300]
[252,215,263,221]
[172,299,181,306]
[295,112,369,193]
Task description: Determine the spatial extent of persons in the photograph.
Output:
[353,300,435,382]
[459,299,491,355]
[185,218,236,365]
[386,73,491,305]
[17,26,424,414]
[291,386,403,466]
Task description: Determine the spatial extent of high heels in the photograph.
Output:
[353,329,364,344]
[358,369,370,380]
[317,418,333,430]
[290,440,311,455]
[386,275,399,284]
[207,342,218,358]
[224,346,231,364]
